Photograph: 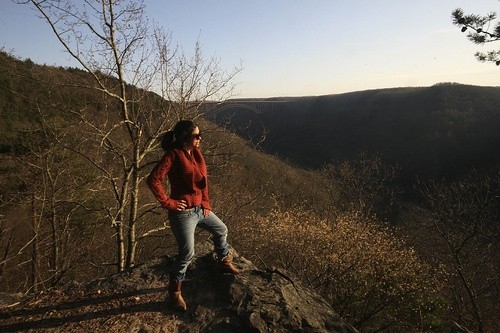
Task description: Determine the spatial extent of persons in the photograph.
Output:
[146,119,245,310]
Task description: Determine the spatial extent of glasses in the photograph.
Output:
[190,134,201,139]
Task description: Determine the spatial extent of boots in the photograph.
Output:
[170,291,186,310]
[218,255,239,274]
